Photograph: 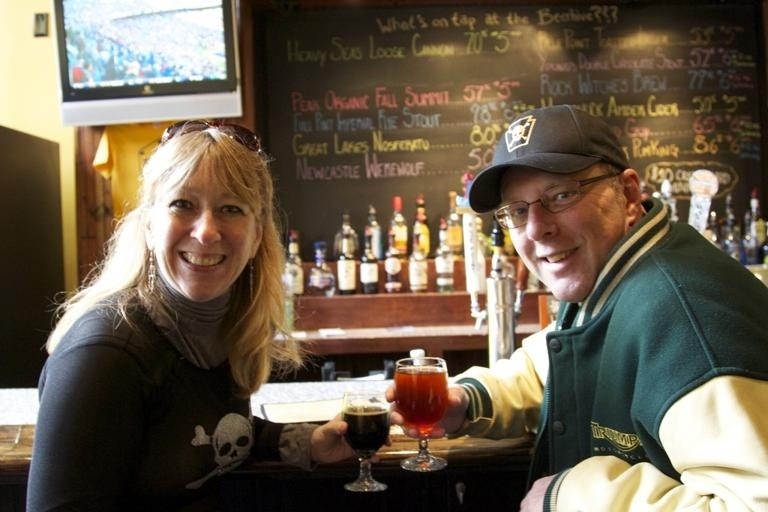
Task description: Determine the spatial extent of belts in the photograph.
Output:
[394,356,449,473]
[341,390,392,493]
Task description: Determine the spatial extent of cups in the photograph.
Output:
[493,174,620,229]
[161,120,261,151]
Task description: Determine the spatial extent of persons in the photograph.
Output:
[387,104,767,512]
[24,117,393,511]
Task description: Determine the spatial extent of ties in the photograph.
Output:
[50,0,244,129]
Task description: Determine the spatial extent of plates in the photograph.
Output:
[278,252,539,367]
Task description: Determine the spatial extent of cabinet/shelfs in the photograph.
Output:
[337,227,357,295]
[405,232,428,293]
[335,188,516,257]
[434,222,453,292]
[357,229,380,296]
[285,230,305,296]
[639,171,765,265]
[383,231,403,294]
[306,240,337,297]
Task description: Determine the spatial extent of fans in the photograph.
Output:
[244,0,768,274]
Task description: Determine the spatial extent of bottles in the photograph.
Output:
[468,104,630,212]
